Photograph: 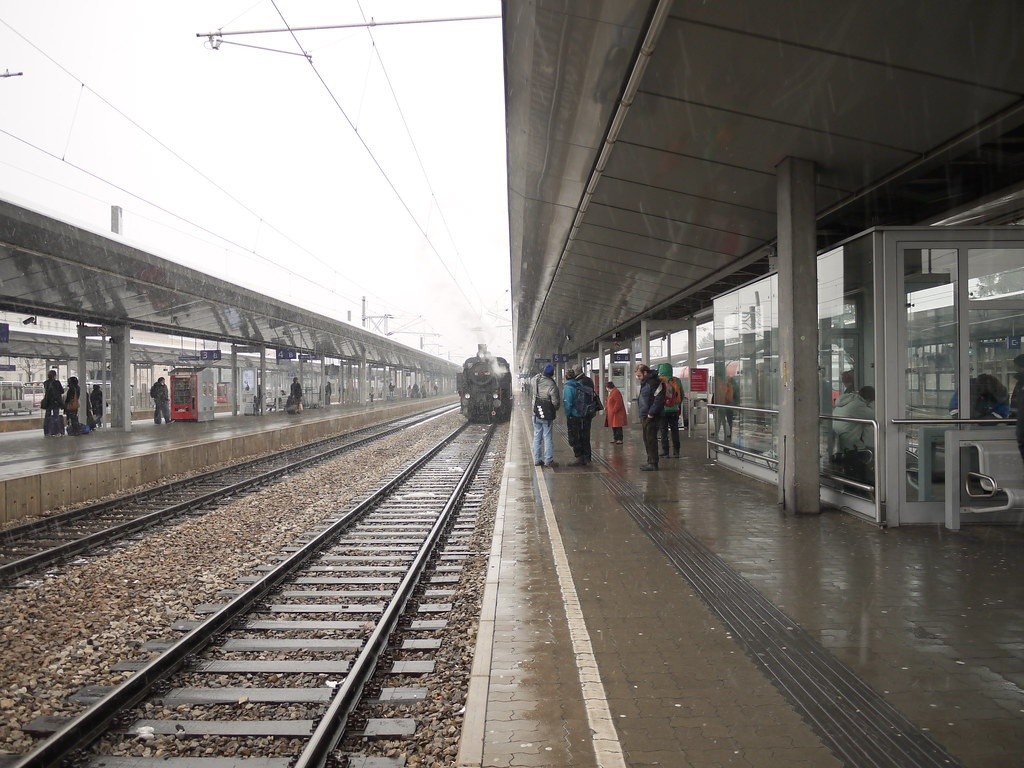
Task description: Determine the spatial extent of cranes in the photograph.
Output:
[361,295,451,358]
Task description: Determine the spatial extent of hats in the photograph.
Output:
[544,363,555,376]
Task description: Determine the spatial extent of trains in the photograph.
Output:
[455,343,513,423]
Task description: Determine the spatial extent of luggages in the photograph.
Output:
[44,414,65,436]
[286,394,298,414]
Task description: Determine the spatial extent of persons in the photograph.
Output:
[44,370,108,435]
[280,390,285,395]
[950,374,1010,426]
[150,377,176,424]
[521,382,527,392]
[634,364,664,471]
[338,384,345,403]
[531,363,560,467]
[1011,354,1024,409]
[819,367,831,417]
[389,382,395,396]
[574,368,594,462]
[833,385,875,450]
[604,381,628,444]
[325,382,331,403]
[658,363,684,458]
[563,369,586,465]
[286,377,303,415]
[841,370,854,392]
[707,377,740,437]
[408,382,426,398]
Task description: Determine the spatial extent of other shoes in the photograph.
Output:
[659,451,671,459]
[671,449,680,459]
[639,461,659,471]
[568,456,584,466]
[610,439,617,444]
[545,460,559,468]
[616,440,623,444]
[584,457,590,465]
[534,460,544,467]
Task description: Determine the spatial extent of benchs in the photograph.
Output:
[957,439,1024,513]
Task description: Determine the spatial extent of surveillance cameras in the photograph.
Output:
[23,317,35,325]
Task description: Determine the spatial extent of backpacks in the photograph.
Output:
[149,386,157,398]
[566,382,598,419]
[661,377,681,407]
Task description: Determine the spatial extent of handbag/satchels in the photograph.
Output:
[66,424,91,435]
[67,397,80,411]
[40,396,49,410]
[533,396,557,421]
[603,415,609,427]
[593,395,605,411]
